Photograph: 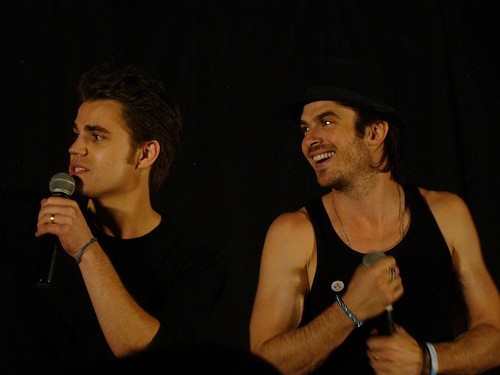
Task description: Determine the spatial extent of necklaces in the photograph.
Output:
[332,182,403,249]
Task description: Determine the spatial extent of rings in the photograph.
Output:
[50,213,55,224]
[391,268,396,280]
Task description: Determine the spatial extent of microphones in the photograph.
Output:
[361,249,393,338]
[35,172,75,289]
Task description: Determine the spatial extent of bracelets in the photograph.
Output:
[336,294,363,327]
[77,239,97,264]
[419,339,438,375]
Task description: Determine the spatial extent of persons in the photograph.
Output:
[248,54,500,375]
[0,74,244,375]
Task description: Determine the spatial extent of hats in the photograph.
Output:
[287,60,407,132]
